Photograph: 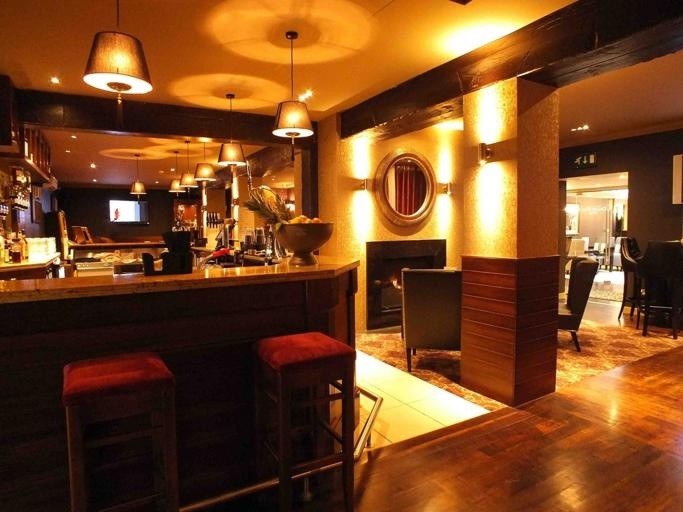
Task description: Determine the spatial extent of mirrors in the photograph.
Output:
[375,147,438,228]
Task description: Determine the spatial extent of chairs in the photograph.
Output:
[565,239,584,274]
[401,267,464,373]
[558,257,599,352]
[582,237,627,271]
[618,238,641,322]
[636,240,683,340]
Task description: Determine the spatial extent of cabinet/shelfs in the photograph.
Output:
[0,252,61,280]
[0,124,53,188]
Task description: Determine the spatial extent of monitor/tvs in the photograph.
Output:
[106,198,149,226]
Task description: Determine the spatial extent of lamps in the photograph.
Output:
[218,93,246,184]
[129,154,147,204]
[82,0,153,125]
[179,140,199,193]
[272,30,314,162]
[168,151,186,197]
[477,142,490,163]
[194,142,217,205]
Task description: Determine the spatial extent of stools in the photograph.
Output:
[252,331,354,511]
[63,352,179,512]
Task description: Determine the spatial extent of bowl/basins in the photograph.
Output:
[271,222,334,266]
